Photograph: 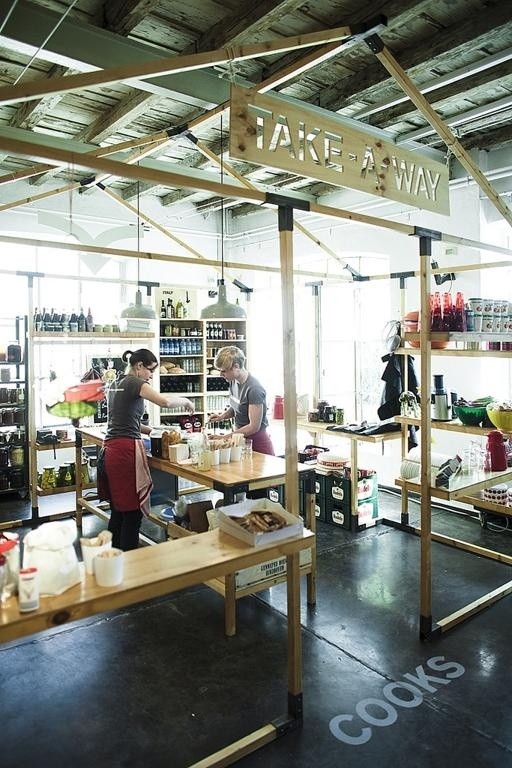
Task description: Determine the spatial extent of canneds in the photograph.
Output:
[94,324,121,332]
[186,382,200,392]
[180,359,200,373]
[222,328,245,340]
[7,340,21,362]
[308,399,343,425]
[207,347,221,357]
[0,388,25,490]
[40,461,90,489]
[465,298,512,332]
[150,426,181,460]
[164,323,202,337]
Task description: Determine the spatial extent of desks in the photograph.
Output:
[75,426,317,636]
[269,414,411,532]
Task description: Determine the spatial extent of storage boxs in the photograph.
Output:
[267,454,378,532]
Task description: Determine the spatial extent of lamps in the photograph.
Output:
[201,111,248,322]
[120,161,157,322]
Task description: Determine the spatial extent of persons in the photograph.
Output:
[96,349,196,552]
[207,347,276,500]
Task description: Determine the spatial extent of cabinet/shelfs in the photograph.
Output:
[149,317,250,429]
[394,330,512,516]
[31,330,155,496]
[0,315,31,500]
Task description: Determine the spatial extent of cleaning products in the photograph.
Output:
[198,427,213,470]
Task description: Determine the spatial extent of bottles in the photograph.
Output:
[160,298,236,358]
[17,566,40,613]
[33,306,121,333]
[0,340,27,490]
[307,398,345,425]
[429,292,475,332]
[40,461,91,490]
[483,429,508,472]
[273,395,284,419]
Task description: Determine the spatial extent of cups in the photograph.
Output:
[94,548,124,588]
[197,446,242,472]
[80,537,112,575]
